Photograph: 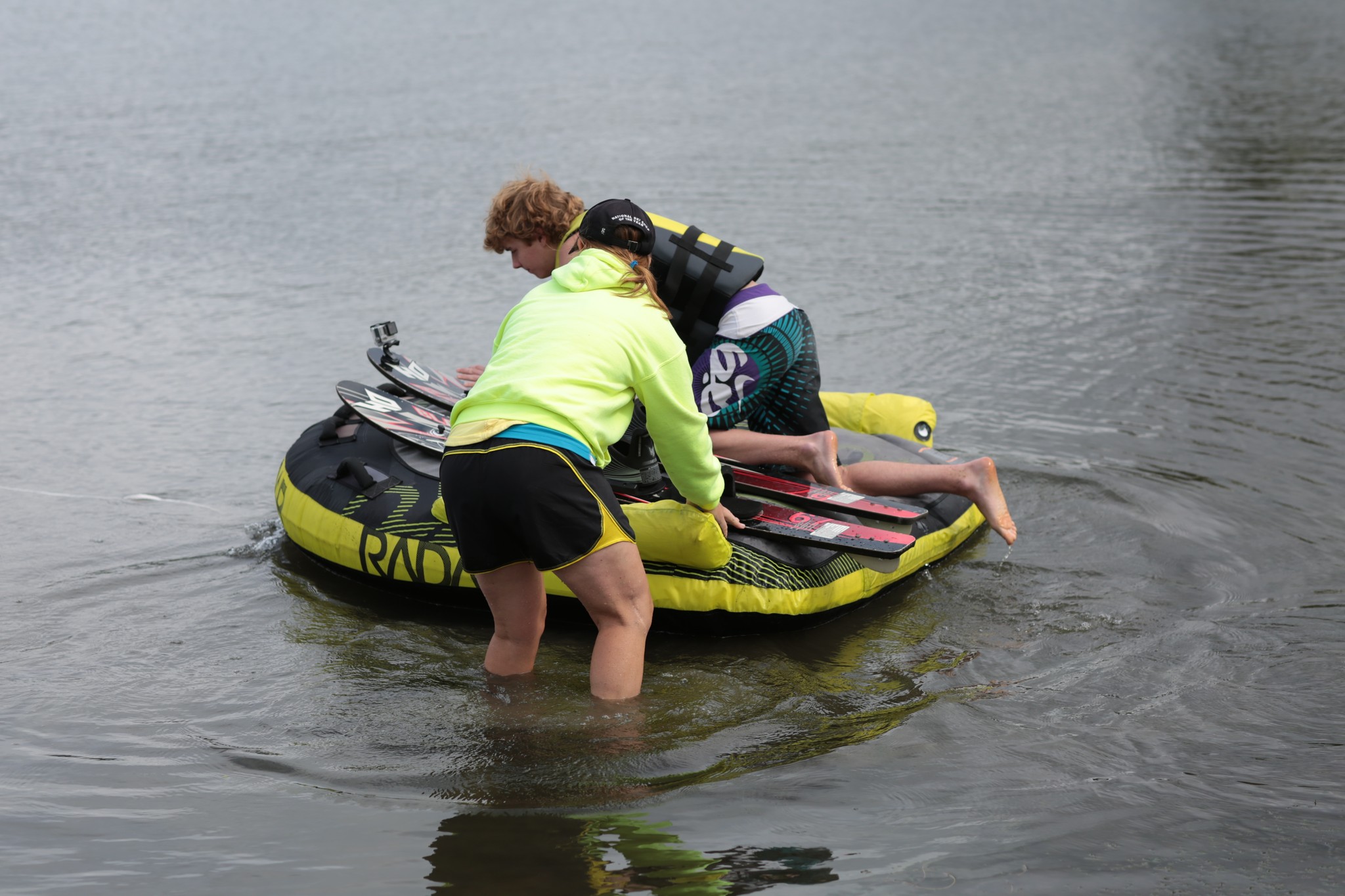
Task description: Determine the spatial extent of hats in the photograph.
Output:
[569,199,656,256]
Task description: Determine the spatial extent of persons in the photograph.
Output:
[453,173,1019,547]
[438,197,745,699]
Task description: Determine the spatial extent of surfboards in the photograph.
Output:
[336,321,929,556]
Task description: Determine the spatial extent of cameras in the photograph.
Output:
[371,321,397,348]
